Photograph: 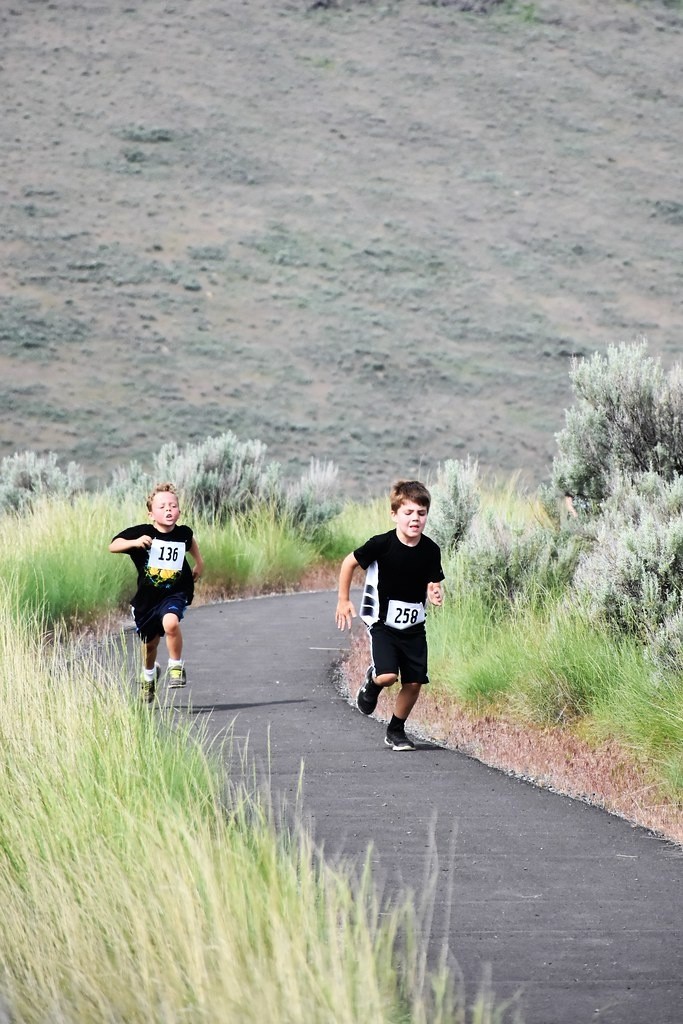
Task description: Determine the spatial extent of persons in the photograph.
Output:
[334,480,445,750]
[108,482,203,704]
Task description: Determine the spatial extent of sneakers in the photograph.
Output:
[355,666,382,715]
[167,663,187,688]
[384,724,416,750]
[139,661,161,703]
